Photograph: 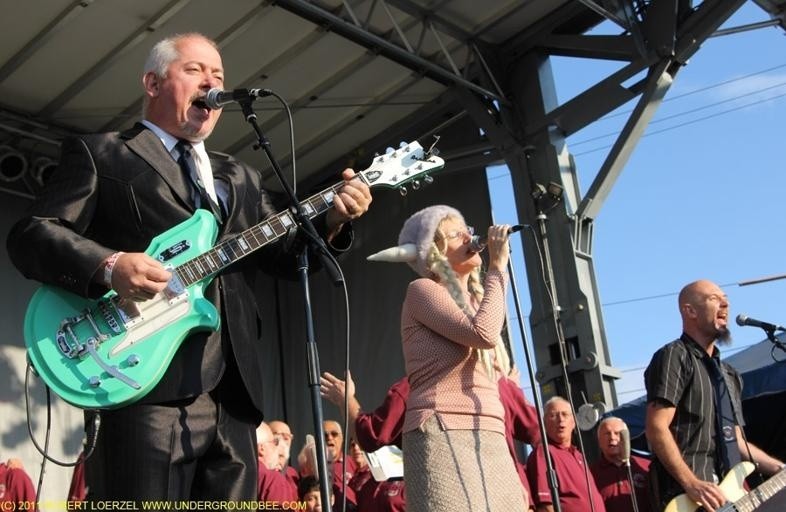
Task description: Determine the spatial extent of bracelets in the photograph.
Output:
[103,251,125,284]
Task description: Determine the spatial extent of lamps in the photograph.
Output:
[0,140,58,189]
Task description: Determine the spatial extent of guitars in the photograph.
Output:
[665,461,785,511]
[23,140,445,409]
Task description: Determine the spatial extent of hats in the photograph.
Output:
[366,204,465,278]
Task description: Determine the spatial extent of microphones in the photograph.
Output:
[469,223,530,253]
[736,313,786,332]
[205,87,270,111]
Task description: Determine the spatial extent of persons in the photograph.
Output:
[4,31,374,512]
[642,279,786,511]
[0,462,39,512]
[6,457,24,470]
[365,204,533,511]
[64,362,653,512]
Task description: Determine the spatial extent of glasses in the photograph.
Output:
[324,430,341,438]
[435,226,476,246]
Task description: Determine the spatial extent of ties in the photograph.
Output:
[706,358,742,469]
[174,139,203,211]
